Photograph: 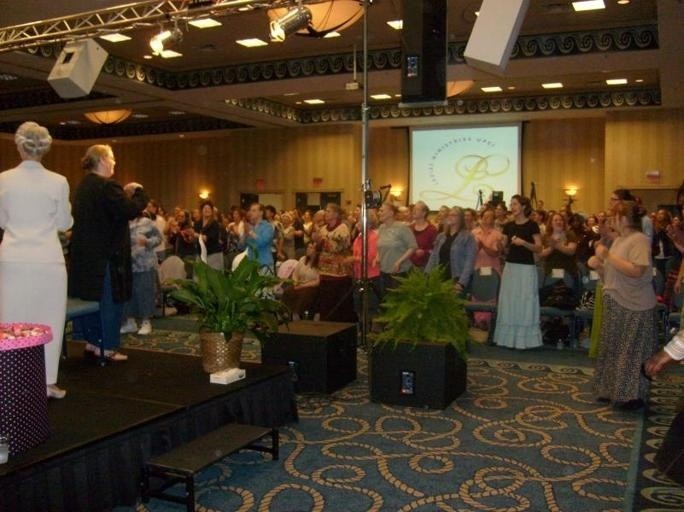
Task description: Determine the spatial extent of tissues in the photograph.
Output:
[210,367,246,385]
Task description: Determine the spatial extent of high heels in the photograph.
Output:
[45,385,67,399]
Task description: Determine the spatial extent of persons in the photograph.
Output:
[120,180,595,348]
[585,185,683,487]
[65,142,133,367]
[0,121,75,399]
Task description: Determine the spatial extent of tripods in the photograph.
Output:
[529,189,537,209]
[476,196,483,211]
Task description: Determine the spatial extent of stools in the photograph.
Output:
[61,298,106,366]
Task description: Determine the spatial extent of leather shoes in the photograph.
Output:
[85,341,101,356]
[99,347,130,362]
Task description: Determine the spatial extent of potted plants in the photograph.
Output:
[366,262,475,412]
[167,254,297,376]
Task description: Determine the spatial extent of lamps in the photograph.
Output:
[560,185,580,215]
[267,6,313,43]
[148,28,183,54]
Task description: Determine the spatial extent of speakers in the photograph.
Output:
[369,339,466,411]
[263,319,359,394]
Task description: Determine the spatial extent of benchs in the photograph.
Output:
[571,308,669,355]
[139,421,279,512]
[460,299,575,355]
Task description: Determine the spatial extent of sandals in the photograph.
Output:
[597,397,612,406]
[615,397,644,412]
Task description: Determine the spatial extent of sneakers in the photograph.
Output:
[138,321,154,335]
[120,322,138,335]
